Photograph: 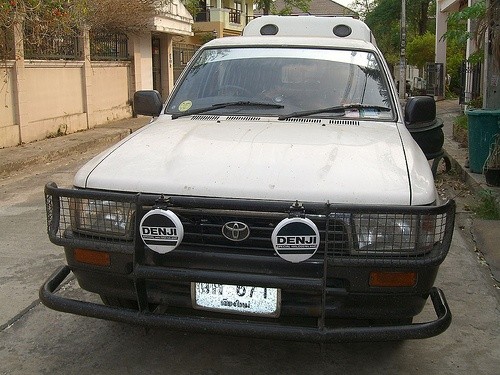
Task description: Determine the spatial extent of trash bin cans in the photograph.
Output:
[465,109,500,174]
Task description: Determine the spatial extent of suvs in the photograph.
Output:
[39,11,458,343]
